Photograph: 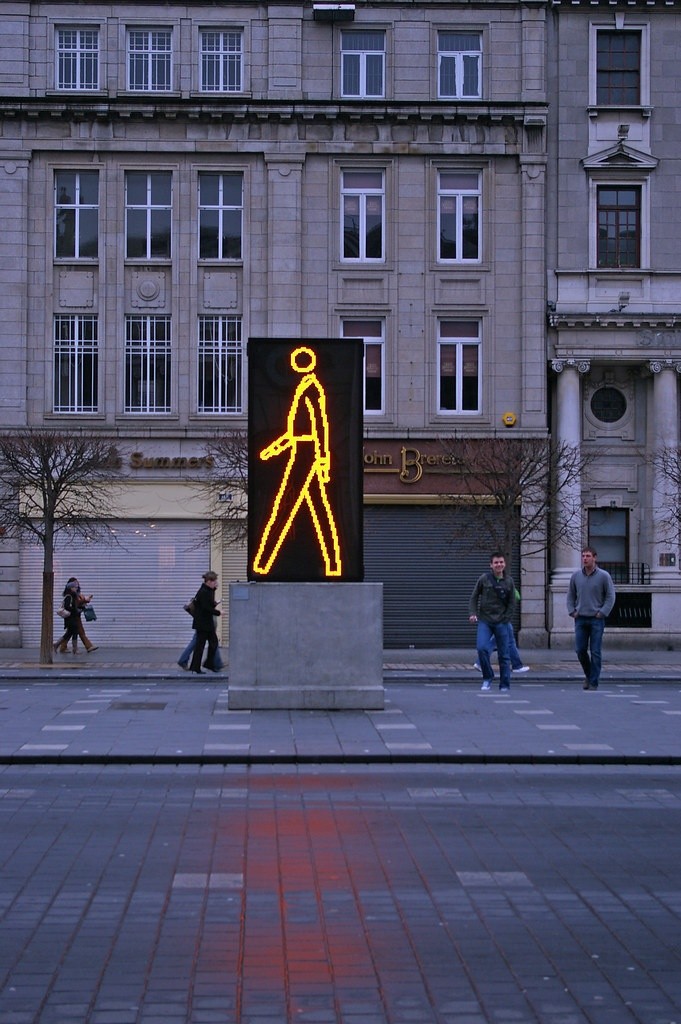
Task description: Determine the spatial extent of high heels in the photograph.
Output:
[203,663,219,672]
[190,667,206,674]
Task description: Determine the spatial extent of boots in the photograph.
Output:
[53,637,65,654]
[72,639,82,654]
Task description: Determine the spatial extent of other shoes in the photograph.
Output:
[60,648,71,653]
[178,661,190,671]
[220,664,229,668]
[481,679,492,690]
[582,676,590,689]
[500,684,508,691]
[512,665,529,672]
[589,684,597,691]
[87,646,99,652]
[473,663,482,672]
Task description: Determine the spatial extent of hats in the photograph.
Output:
[66,582,78,589]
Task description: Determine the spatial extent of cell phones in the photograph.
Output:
[90,595,93,599]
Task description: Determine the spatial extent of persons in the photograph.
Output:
[566,546,616,690]
[53,577,99,654]
[469,552,529,692]
[178,571,228,674]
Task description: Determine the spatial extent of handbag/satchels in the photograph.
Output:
[57,594,72,618]
[183,598,195,617]
[82,601,97,620]
